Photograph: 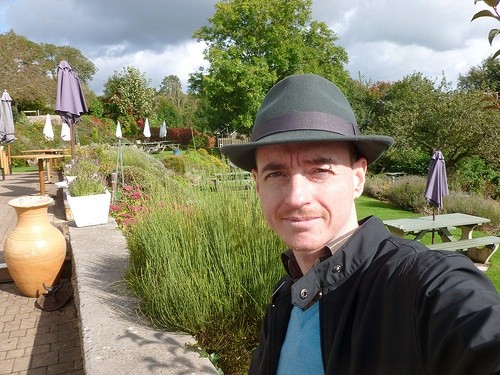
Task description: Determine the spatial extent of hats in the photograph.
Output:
[219,74,394,172]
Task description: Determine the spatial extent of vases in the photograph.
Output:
[3,195,66,299]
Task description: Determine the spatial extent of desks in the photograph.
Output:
[382,211,491,246]
[7,146,67,198]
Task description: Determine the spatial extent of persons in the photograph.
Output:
[220,75,500,375]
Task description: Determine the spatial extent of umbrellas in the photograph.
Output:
[424,151,449,244]
[55,60,88,159]
[61,121,71,141]
[143,117,151,138]
[159,122,167,138]
[0,91,15,180]
[43,113,53,150]
[116,121,122,139]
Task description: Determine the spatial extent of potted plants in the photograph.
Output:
[67,140,116,226]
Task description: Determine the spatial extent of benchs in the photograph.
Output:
[422,236,500,272]
